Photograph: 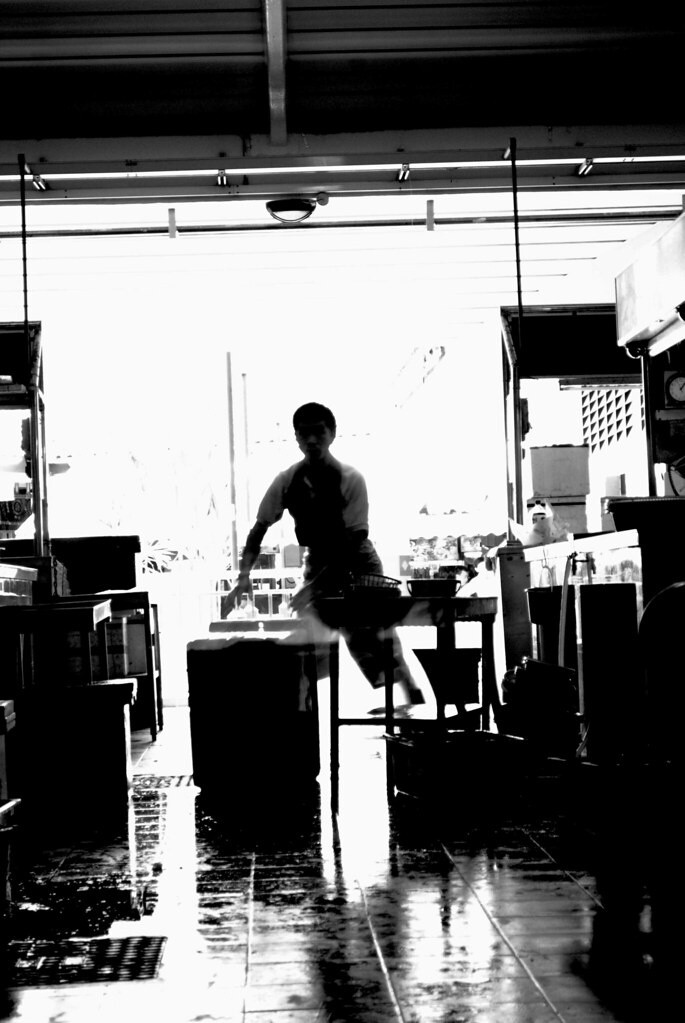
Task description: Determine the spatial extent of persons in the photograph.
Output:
[224,401,424,706]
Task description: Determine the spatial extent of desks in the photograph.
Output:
[319,597,498,817]
[0,599,113,690]
[61,591,163,742]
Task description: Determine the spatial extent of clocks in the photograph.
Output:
[666,370,685,408]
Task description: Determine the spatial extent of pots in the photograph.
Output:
[408,579,462,596]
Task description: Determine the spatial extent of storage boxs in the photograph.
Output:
[0,535,141,594]
[51,617,128,679]
[382,729,525,798]
[127,607,156,675]
